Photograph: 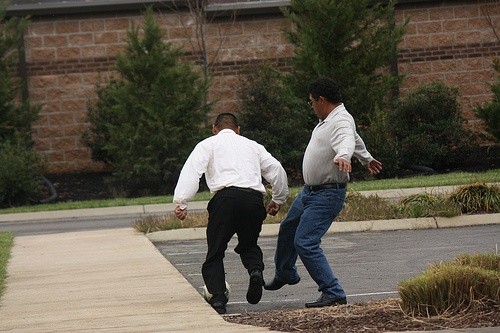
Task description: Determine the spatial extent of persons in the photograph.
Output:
[173,113,289,314]
[264,77,383,308]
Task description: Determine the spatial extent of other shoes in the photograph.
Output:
[246,269,263,305]
[211,300,226,314]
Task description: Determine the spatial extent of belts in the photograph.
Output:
[309,183,346,192]
[217,186,263,198]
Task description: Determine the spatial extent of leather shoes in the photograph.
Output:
[305,293,347,308]
[264,276,301,290]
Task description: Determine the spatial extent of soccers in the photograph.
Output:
[201,281,231,306]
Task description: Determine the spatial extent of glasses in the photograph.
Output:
[307,98,318,108]
